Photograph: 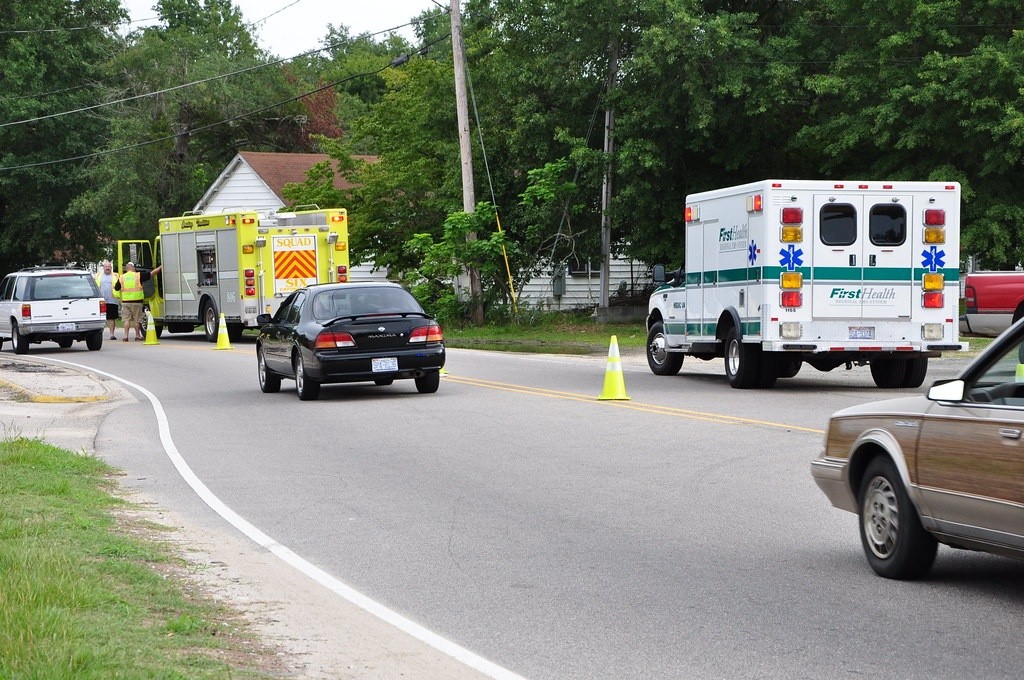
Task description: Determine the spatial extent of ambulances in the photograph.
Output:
[645,179,967,390]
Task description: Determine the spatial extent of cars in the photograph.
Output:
[809,317,1024,581]
[256,282,445,401]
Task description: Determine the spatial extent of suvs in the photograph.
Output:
[0,265,108,355]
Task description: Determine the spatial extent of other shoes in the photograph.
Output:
[135,337,146,341]
[109,336,117,340]
[122,337,128,342]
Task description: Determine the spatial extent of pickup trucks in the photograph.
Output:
[959,252,1024,338]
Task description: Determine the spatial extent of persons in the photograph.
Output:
[115,262,161,342]
[94,262,121,340]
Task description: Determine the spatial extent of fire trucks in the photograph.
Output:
[117,203,351,344]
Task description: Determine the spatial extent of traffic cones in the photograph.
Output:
[143,311,161,346]
[597,335,632,400]
[210,312,236,350]
